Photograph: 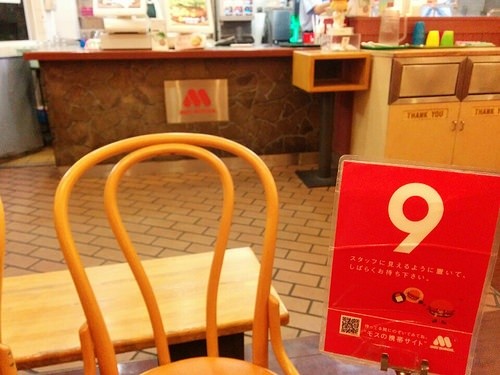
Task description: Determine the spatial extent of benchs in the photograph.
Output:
[4,245,289,370]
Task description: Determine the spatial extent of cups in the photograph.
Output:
[413,22,425,46]
[426,30,439,47]
[441,31,454,47]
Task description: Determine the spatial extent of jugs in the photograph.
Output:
[379,8,407,46]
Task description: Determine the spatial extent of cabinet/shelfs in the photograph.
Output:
[350,47,500,173]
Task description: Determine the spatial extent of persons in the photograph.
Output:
[299,0,330,33]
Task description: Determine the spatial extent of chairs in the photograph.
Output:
[54,132,300,375]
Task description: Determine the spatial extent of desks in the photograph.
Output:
[292,50,371,188]
